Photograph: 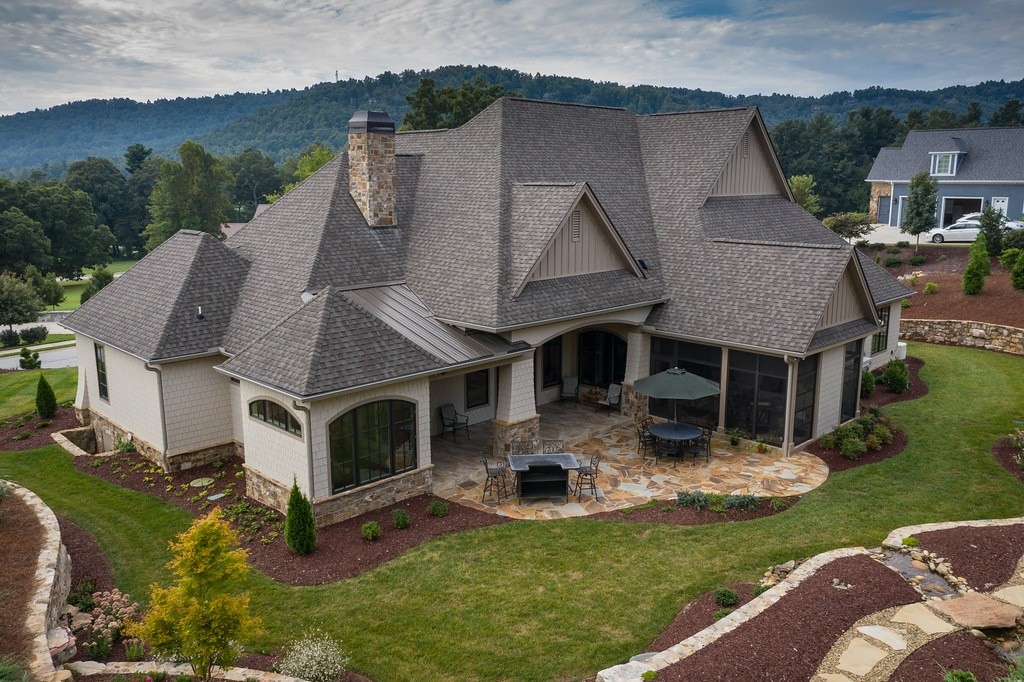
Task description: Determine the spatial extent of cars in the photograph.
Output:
[925,221,982,244]
[956,213,1024,234]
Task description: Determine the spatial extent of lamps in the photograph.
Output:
[984,200,989,207]
[893,197,897,205]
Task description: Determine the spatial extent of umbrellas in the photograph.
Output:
[634,367,721,423]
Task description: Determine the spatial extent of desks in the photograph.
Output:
[581,392,606,407]
[508,452,580,505]
[649,422,704,460]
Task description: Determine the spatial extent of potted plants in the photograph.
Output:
[726,427,749,447]
[749,435,767,453]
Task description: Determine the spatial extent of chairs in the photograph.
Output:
[573,449,602,503]
[543,439,573,494]
[559,375,579,408]
[440,403,470,442]
[480,455,508,505]
[595,383,621,417]
[511,440,534,494]
[637,417,715,467]
